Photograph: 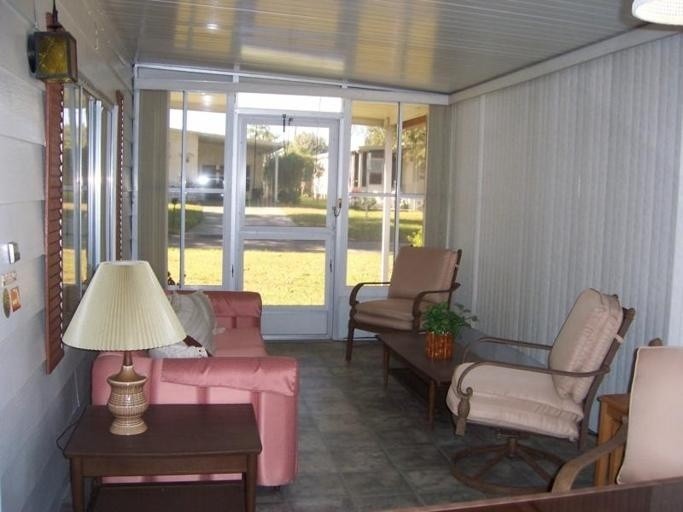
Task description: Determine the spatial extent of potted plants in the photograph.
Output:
[409,299,480,361]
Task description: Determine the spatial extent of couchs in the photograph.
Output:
[89,288,299,495]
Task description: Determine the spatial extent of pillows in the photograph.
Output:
[148,288,226,358]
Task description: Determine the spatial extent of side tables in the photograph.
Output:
[62,403,263,511]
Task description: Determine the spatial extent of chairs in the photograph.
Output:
[344,244,462,364]
[551,346,683,490]
[448,288,635,497]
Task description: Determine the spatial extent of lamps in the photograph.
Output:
[61,259,188,436]
[26,31,79,84]
